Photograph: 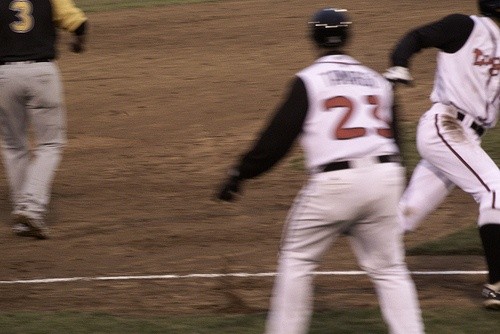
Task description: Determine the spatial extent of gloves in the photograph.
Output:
[66,20,89,55]
[215,164,243,204]
[381,68,416,90]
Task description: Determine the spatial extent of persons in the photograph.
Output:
[385,1,499,306]
[0,0,88,241]
[214,6,425,334]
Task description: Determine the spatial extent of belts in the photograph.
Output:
[315,155,401,174]
[456,111,487,139]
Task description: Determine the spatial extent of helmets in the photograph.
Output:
[308,9,350,49]
[477,0,500,15]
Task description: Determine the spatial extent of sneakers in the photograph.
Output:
[482,283,500,310]
[11,214,51,240]
